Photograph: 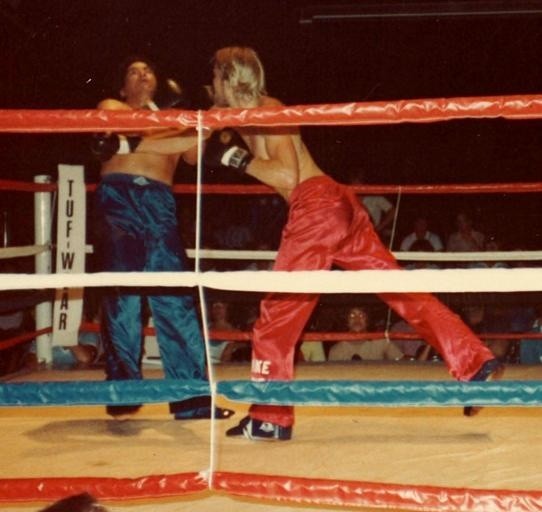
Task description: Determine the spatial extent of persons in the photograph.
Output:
[184,210,542,272]
[93,296,541,364]
[88,59,234,423]
[89,46,506,439]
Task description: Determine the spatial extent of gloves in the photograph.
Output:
[217,143,253,172]
[148,80,181,109]
[89,131,139,157]
